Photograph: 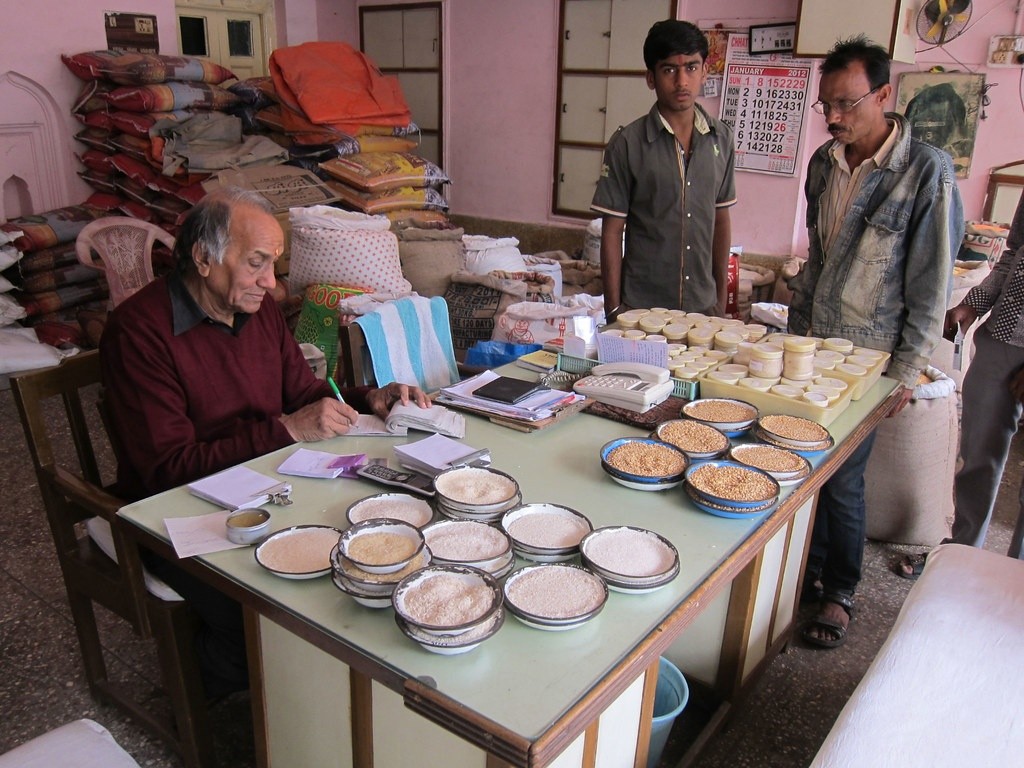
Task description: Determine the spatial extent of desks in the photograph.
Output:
[114,373,905,768]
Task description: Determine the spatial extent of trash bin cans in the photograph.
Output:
[647,656,689,768]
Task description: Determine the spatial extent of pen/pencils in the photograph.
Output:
[327,377,358,428]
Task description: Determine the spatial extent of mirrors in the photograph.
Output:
[980,159,1024,226]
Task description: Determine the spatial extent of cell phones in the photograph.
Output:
[355,465,436,500]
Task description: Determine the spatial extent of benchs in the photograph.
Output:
[808,543,1024,768]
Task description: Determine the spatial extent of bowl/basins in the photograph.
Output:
[225,508,271,545]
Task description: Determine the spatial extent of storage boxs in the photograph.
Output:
[558,333,891,428]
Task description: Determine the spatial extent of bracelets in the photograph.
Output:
[604,306,619,319]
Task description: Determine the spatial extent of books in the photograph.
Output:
[345,399,466,438]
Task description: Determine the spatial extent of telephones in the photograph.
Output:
[574,362,675,413]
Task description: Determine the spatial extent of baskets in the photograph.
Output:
[670,376,701,402]
[556,353,606,377]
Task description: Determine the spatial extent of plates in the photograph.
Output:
[420,517,516,581]
[579,525,681,595]
[346,493,435,531]
[726,443,813,486]
[683,460,781,519]
[753,414,834,458]
[501,502,595,563]
[649,420,732,463]
[255,524,342,579]
[679,399,760,438]
[600,437,692,492]
[504,563,610,632]
[433,467,522,525]
[392,564,504,655]
[329,518,433,608]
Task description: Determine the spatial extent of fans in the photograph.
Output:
[915,0,974,47]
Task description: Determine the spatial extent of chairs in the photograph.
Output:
[9,346,241,768]
[75,216,176,311]
[338,295,497,394]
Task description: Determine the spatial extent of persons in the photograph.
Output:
[786,38,965,648]
[589,19,737,325]
[95,184,432,698]
[895,188,1024,579]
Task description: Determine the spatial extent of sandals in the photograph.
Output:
[799,591,857,649]
[896,553,927,580]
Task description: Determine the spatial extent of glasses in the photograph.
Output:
[810,86,885,115]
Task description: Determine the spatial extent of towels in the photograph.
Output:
[350,295,461,395]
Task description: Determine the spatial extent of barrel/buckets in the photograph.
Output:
[646,655,689,768]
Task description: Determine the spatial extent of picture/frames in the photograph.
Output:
[749,22,795,55]
